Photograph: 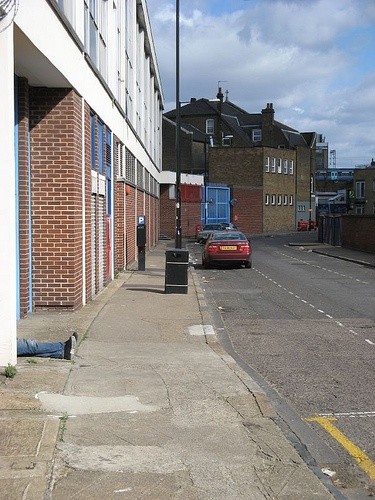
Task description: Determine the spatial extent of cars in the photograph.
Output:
[220,223,238,230]
[199,230,253,269]
[199,223,226,243]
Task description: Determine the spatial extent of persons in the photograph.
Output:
[17,332,79,360]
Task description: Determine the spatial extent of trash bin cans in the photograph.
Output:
[165,248,189,294]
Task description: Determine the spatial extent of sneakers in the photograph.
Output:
[63,332,78,361]
